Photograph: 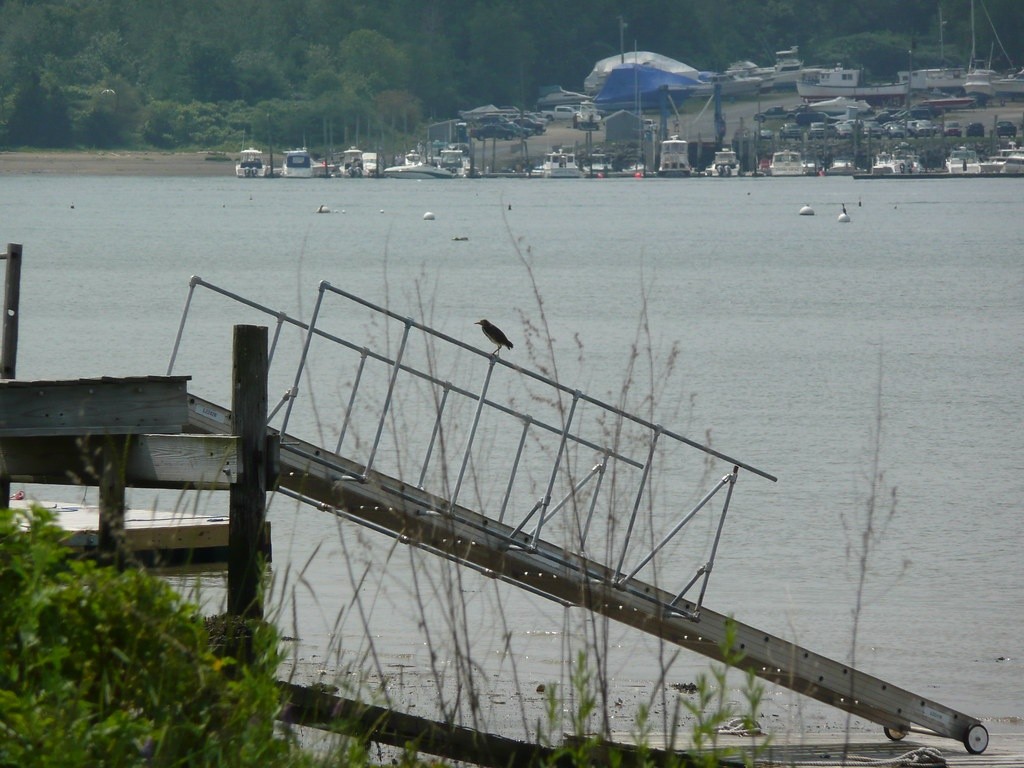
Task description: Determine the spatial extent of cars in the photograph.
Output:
[470,125,516,140]
[942,121,962,136]
[807,122,827,140]
[964,123,985,137]
[990,121,1017,138]
[827,104,943,139]
[476,112,546,140]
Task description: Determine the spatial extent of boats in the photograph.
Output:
[234,128,271,178]
[383,142,470,181]
[282,132,318,180]
[656,135,692,178]
[335,147,383,179]
[707,147,740,177]
[795,64,909,105]
[808,141,1024,176]
[538,148,582,179]
[923,97,976,110]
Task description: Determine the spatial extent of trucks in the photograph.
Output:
[770,148,810,177]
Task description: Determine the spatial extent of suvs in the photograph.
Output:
[779,123,803,140]
[797,112,838,126]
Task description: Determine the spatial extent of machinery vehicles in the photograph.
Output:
[573,100,602,131]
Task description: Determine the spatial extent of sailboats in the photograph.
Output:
[899,0,1024,99]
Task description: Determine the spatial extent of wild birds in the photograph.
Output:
[472,318,514,358]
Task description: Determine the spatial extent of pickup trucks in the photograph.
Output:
[753,106,797,122]
[542,106,577,120]
